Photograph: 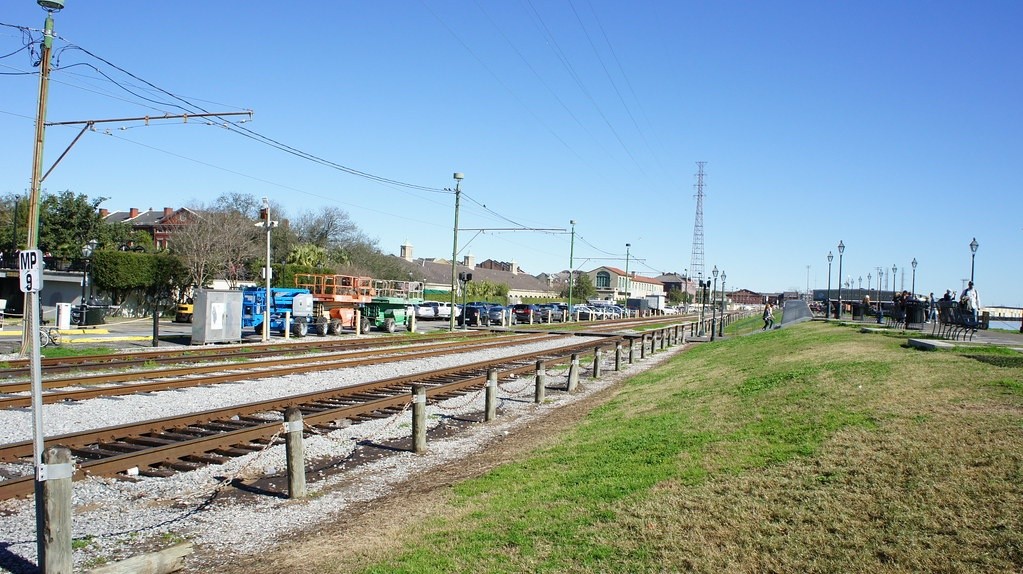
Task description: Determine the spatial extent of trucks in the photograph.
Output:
[626,299,650,317]
[646,294,666,315]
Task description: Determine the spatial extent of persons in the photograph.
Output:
[762,303,774,331]
[808,281,980,332]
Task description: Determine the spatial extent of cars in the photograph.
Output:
[415,299,625,327]
[665,304,708,314]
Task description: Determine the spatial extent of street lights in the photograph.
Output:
[851,278,854,305]
[858,276,862,304]
[867,273,872,295]
[911,258,917,299]
[458,272,473,330]
[891,264,897,292]
[878,269,883,312]
[836,239,845,319]
[719,270,726,338]
[710,265,719,342]
[969,237,979,281]
[826,251,834,319]
[79,238,99,326]
[254,195,279,341]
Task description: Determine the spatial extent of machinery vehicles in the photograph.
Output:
[174,274,425,336]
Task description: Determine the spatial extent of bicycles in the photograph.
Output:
[39,326,62,348]
